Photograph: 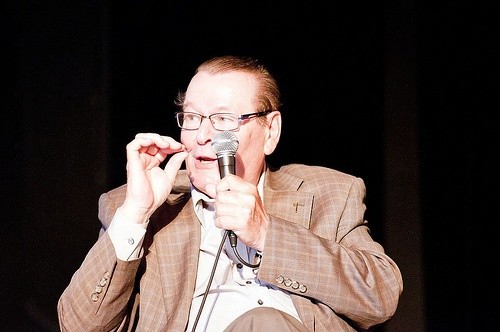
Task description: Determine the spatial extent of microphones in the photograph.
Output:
[211,131,240,247]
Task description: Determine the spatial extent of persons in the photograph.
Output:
[55,59,402,332]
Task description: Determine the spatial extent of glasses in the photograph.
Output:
[176,110,272,131]
